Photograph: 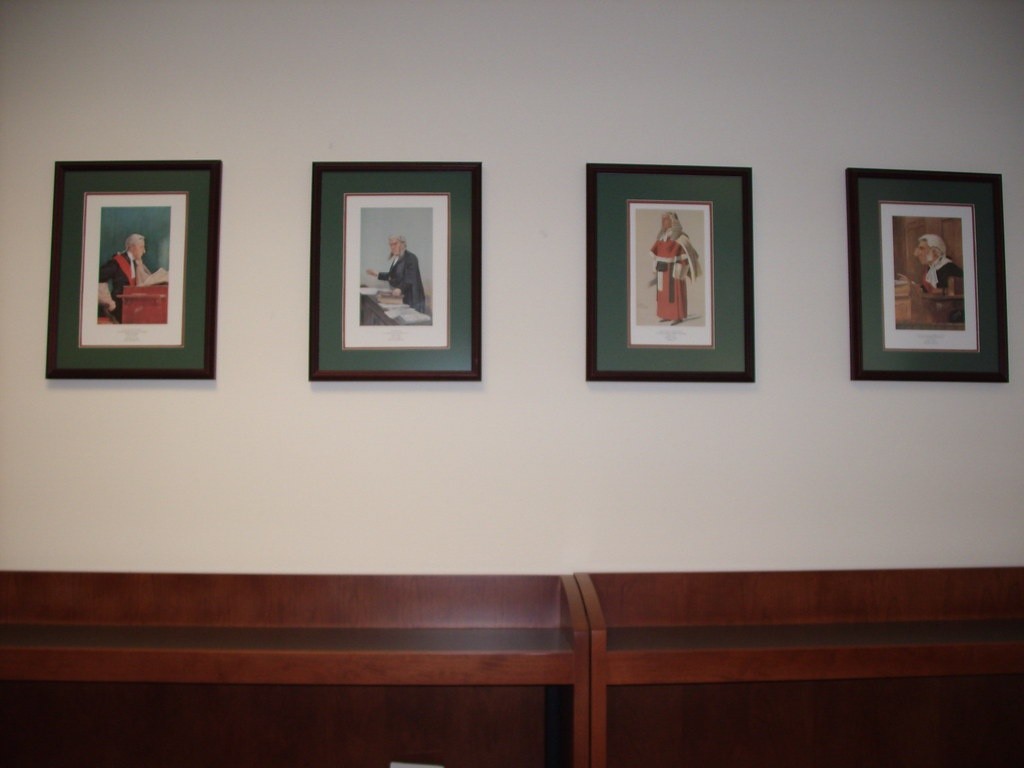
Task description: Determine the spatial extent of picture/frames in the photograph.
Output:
[583,160,756,385]
[842,167,1011,384]
[45,158,225,382]
[309,158,484,381]
